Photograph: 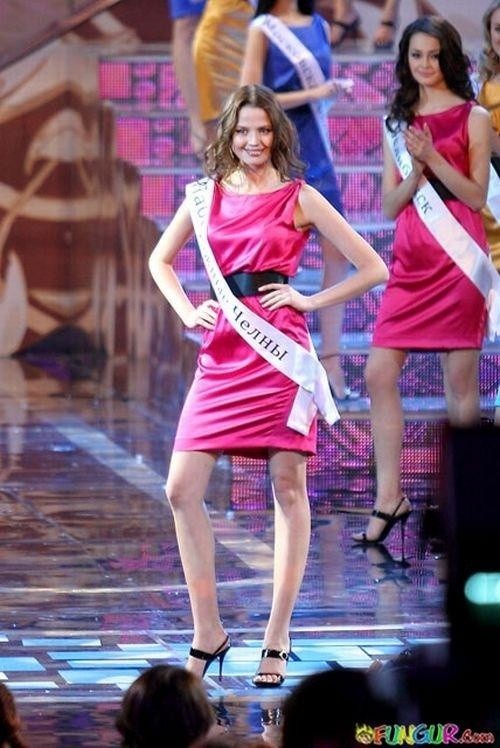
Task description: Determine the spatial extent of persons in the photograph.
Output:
[3,1,500,748]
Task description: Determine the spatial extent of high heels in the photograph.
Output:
[254,636,292,687]
[351,495,413,544]
[354,545,413,585]
[190,634,231,680]
